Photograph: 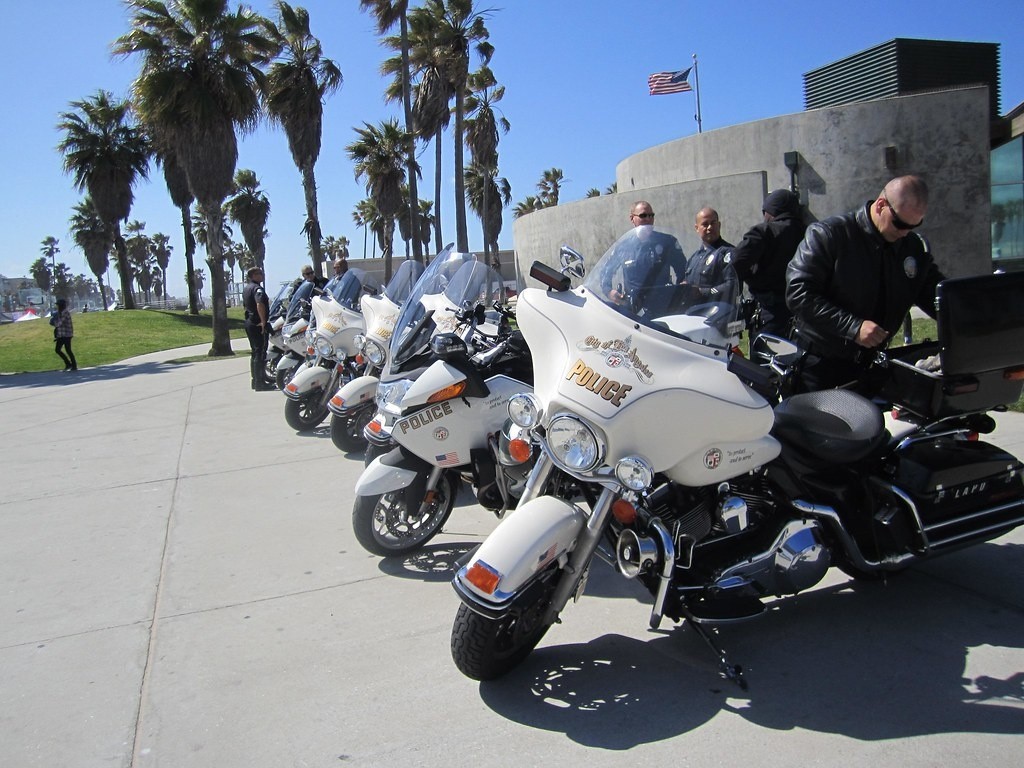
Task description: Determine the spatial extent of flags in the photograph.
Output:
[647,65,694,96]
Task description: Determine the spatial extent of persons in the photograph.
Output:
[678,207,738,303]
[290,266,329,301]
[50,299,78,371]
[600,200,687,308]
[730,189,815,360]
[327,260,360,306]
[242,267,276,392]
[784,175,947,400]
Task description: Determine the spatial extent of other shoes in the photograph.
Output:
[251,378,276,391]
[64,361,77,371]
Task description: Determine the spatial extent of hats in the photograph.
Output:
[762,189,799,218]
[55,299,66,306]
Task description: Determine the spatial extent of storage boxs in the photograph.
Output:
[879,271,1024,420]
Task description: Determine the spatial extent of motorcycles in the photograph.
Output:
[263,242,1024,692]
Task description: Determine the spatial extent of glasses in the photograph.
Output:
[255,272,264,275]
[762,209,767,216]
[303,271,314,275]
[632,213,655,218]
[883,189,924,230]
